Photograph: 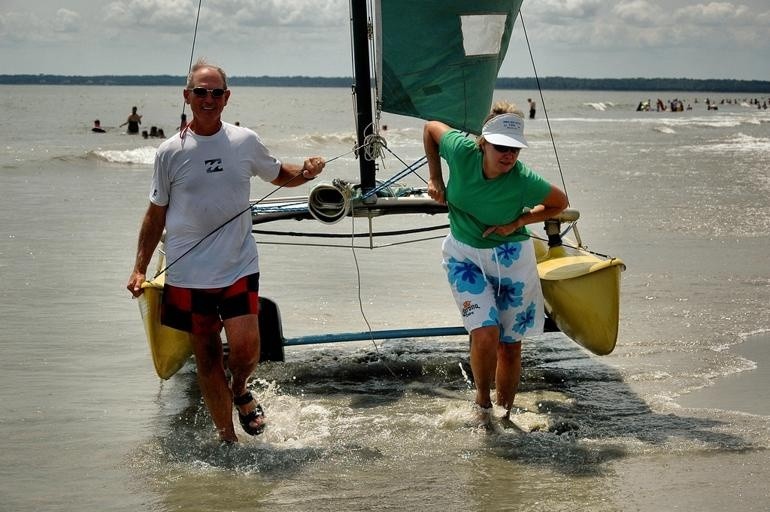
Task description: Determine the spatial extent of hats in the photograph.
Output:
[481,112,528,149]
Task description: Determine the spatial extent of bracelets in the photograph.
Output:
[301,171,315,180]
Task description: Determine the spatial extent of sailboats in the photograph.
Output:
[129,0,624,387]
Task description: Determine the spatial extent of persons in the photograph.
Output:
[636,96,770,113]
[119,106,143,135]
[91,119,106,134]
[422,99,570,437]
[527,98,537,120]
[125,64,326,447]
[177,112,187,133]
[150,126,158,138]
[142,130,148,139]
[157,128,166,137]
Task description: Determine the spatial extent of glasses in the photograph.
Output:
[187,87,226,98]
[492,143,521,154]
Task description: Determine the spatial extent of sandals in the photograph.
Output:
[233,390,266,436]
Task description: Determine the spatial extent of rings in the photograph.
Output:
[316,160,321,166]
